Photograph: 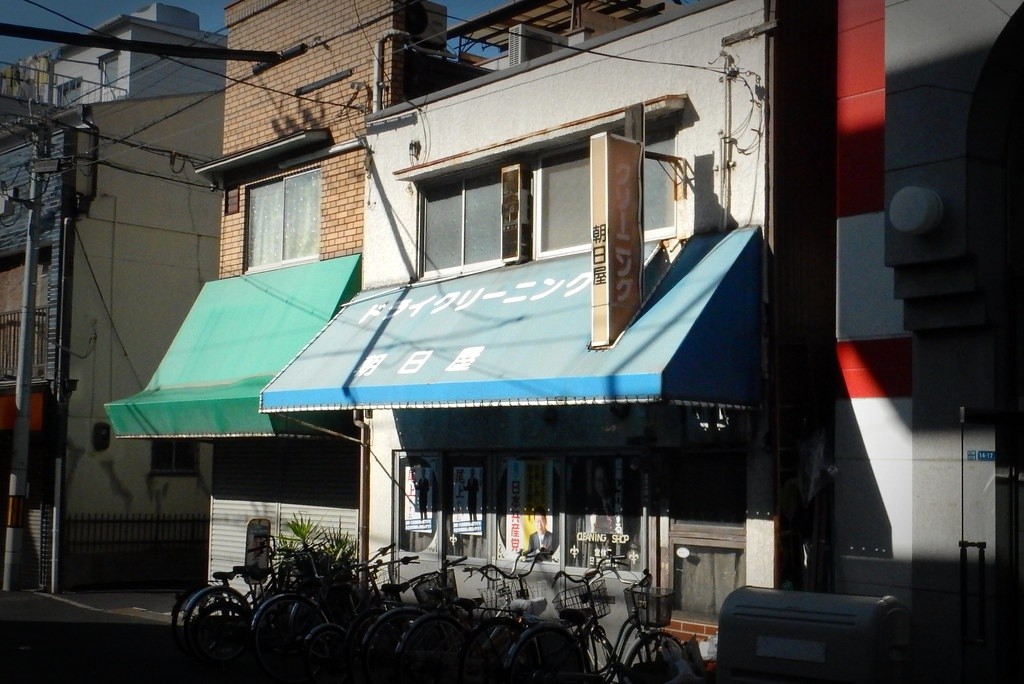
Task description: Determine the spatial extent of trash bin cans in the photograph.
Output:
[716,586,913,684]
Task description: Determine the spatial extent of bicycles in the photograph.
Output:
[171,528,683,684]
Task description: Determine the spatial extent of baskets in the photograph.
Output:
[551,580,613,629]
[478,577,537,617]
[412,569,459,606]
[623,587,674,629]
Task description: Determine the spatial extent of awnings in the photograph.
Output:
[0,392,52,430]
[104,255,359,441]
[258,227,770,445]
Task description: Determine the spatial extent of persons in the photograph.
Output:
[588,465,615,534]
[463,468,479,521]
[414,469,429,519]
[528,508,553,561]
